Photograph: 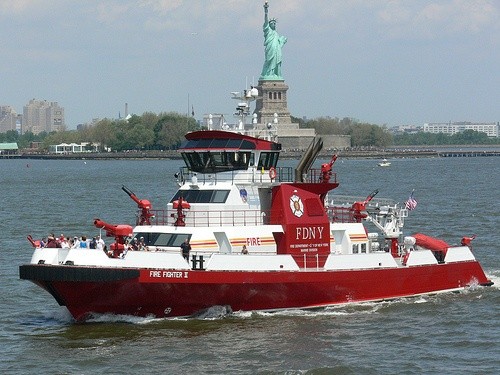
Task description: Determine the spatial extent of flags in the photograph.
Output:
[404,190,418,211]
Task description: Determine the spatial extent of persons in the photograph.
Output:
[39,232,146,258]
[180,238,192,264]
[258,1,288,81]
[240,245,249,255]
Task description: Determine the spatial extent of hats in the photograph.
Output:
[140,237,144,239]
[134,238,137,241]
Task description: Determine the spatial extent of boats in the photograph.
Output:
[379,157,391,167]
[17,75,495,321]
